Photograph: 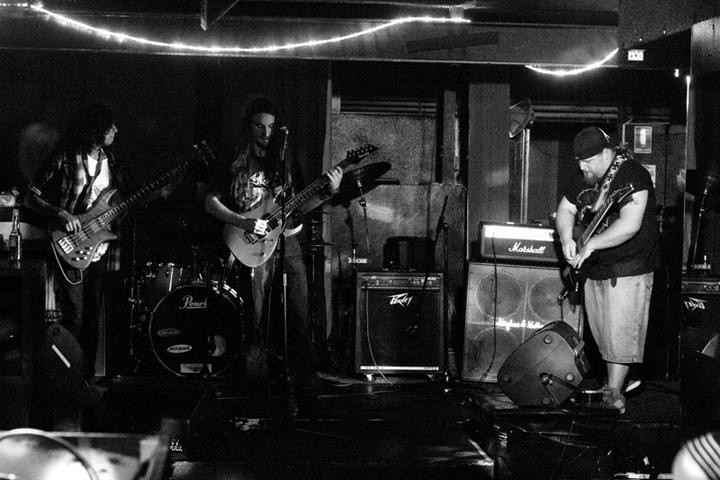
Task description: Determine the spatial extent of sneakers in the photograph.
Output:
[601,388,626,414]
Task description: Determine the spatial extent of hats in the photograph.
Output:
[573,127,613,160]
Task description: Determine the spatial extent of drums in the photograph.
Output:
[147,277,245,378]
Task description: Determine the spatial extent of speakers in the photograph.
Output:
[496,321,591,406]
[356,273,448,374]
[460,262,584,384]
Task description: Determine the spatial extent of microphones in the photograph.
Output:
[277,125,291,163]
[383,236,436,272]
[354,176,366,204]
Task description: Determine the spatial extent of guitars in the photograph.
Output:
[222,143,379,269]
[49,140,217,271]
[553,182,638,305]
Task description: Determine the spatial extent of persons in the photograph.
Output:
[21,93,174,389]
[551,124,662,409]
[202,94,345,420]
